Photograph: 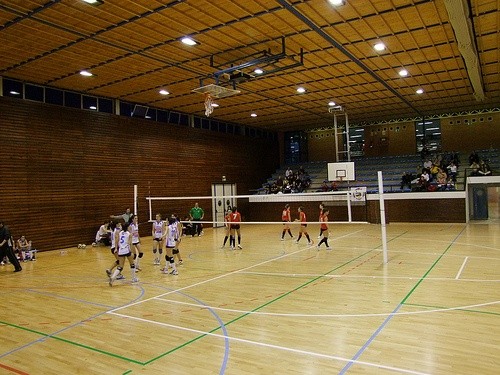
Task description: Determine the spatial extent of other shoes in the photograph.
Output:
[220,245,224,248]
[1,258,35,265]
[230,245,233,248]
[309,241,312,246]
[326,247,332,250]
[132,278,138,282]
[281,238,283,240]
[157,262,160,264]
[170,270,178,274]
[233,247,236,250]
[138,268,142,271]
[177,261,183,264]
[154,262,156,264]
[109,276,114,286]
[317,236,321,239]
[116,274,125,278]
[135,269,138,271]
[316,245,320,251]
[160,268,168,273]
[106,269,112,277]
[14,268,22,272]
[294,240,298,242]
[291,236,295,239]
[238,244,242,248]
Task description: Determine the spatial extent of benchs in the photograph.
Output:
[257,151,500,194]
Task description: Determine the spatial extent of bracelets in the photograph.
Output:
[201,217,203,218]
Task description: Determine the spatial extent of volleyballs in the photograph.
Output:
[91,242,98,247]
[77,243,87,249]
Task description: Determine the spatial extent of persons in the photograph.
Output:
[158,217,180,275]
[127,215,144,272]
[316,209,332,250]
[265,164,312,194]
[319,177,329,192]
[0,234,37,265]
[329,182,338,191]
[468,149,492,176]
[280,204,297,241]
[177,212,204,236]
[109,222,139,286]
[160,212,183,271]
[152,214,167,265]
[99,220,112,247]
[0,221,22,272]
[189,202,204,237]
[295,207,313,245]
[222,206,243,250]
[400,144,460,193]
[109,208,134,227]
[317,203,326,239]
[106,220,125,279]
